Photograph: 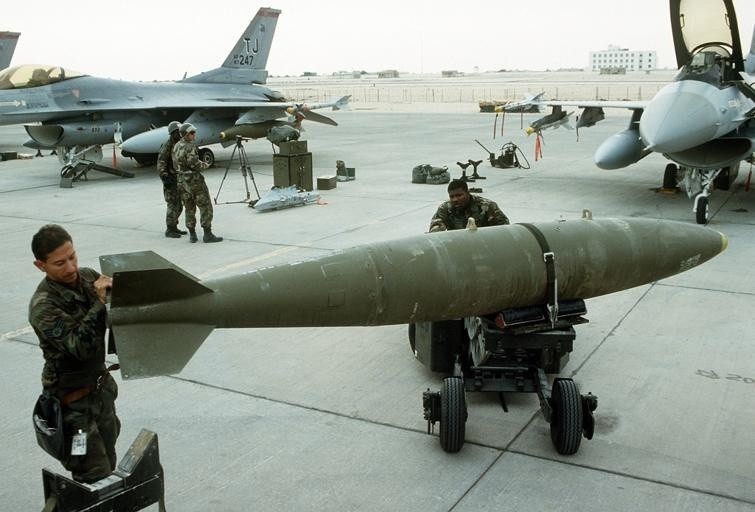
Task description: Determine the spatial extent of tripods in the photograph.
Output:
[214,134,261,204]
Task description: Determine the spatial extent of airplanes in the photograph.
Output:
[481,0,755,225]
[0,7,355,189]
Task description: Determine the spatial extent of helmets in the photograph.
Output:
[179,123,197,136]
[167,120,181,135]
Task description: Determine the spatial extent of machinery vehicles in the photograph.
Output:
[404,295,599,456]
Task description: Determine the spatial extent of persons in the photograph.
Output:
[172,123,222,244]
[29,226,119,479]
[429,179,510,232]
[157,121,187,237]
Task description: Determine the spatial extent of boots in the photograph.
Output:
[165,223,187,238]
[189,228,199,244]
[203,226,223,242]
